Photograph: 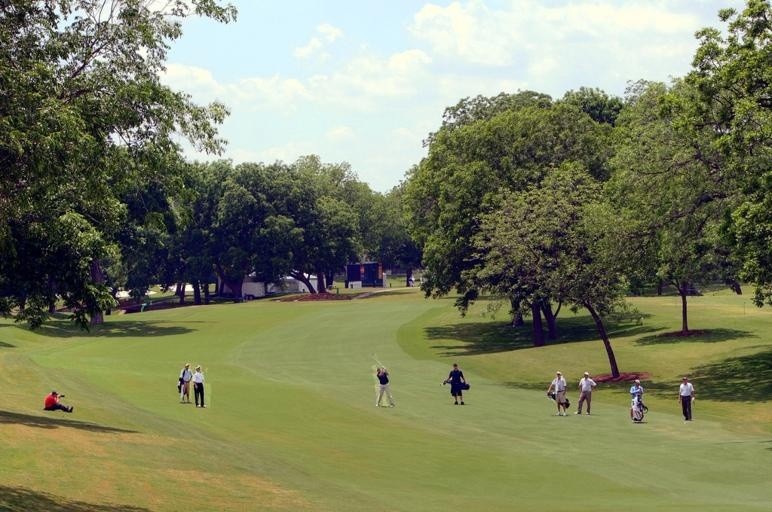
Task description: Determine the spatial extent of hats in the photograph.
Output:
[584,371,590,376]
[635,379,640,384]
[683,377,687,380]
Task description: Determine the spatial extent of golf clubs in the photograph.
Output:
[370,352,390,375]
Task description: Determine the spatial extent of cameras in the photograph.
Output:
[56,394,65,397]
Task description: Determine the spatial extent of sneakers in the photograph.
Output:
[556,412,590,416]
[454,402,464,405]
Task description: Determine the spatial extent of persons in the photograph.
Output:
[546,370,567,416]
[178,363,193,402]
[677,375,695,420]
[574,372,597,415]
[192,366,207,408]
[44,390,74,413]
[375,368,394,407]
[443,363,466,405]
[630,379,643,422]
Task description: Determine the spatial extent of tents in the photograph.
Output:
[216,266,320,298]
